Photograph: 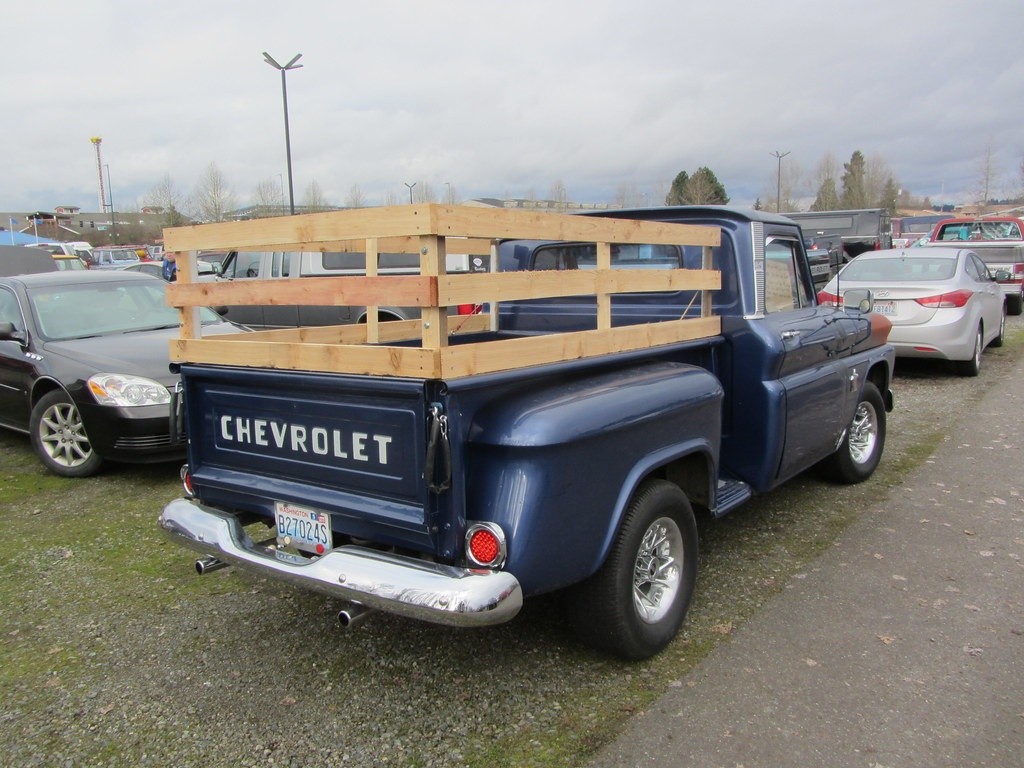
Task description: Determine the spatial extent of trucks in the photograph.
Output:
[776,207,958,256]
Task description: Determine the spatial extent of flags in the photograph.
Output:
[36,218,42,225]
[12,219,18,224]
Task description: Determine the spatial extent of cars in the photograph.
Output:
[0,236,222,296]
[1,265,256,484]
[817,248,1009,372]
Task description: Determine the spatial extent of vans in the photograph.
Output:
[205,225,497,347]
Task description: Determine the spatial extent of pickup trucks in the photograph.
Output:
[915,215,1022,316]
[796,233,844,268]
[800,249,845,301]
[158,199,894,662]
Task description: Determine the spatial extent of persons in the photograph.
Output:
[162,250,178,282]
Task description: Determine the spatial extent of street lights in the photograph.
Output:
[404,183,416,204]
[262,51,305,214]
[770,150,791,213]
